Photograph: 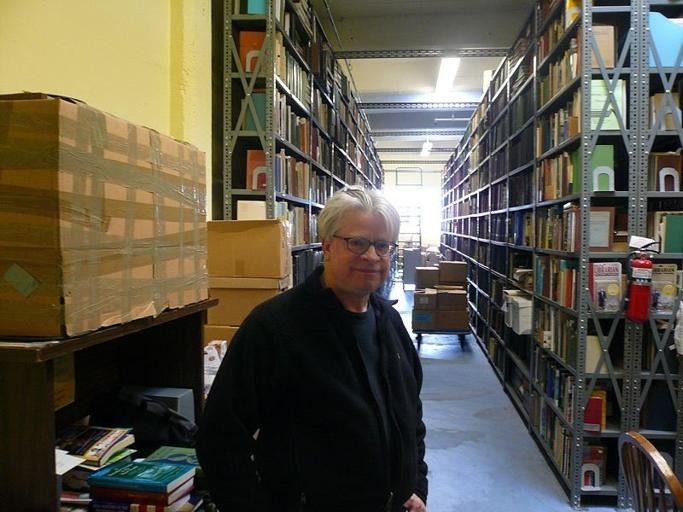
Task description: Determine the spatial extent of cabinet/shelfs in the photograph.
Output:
[0,295,219,510]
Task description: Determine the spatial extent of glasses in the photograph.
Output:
[332,234,400,258]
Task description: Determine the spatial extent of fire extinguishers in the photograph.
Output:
[625,241,661,323]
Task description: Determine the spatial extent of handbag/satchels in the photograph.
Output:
[90,386,203,457]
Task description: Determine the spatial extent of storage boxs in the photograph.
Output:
[441,3,679,495]
[402,244,471,333]
[0,86,209,340]
[204,1,383,347]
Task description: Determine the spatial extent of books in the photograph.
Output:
[57,421,215,511]
[293,249,323,287]
[469,306,477,328]
[237,0,385,206]
[442,0,683,313]
[477,299,683,488]
[238,201,321,246]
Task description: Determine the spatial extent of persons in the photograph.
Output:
[200,187,429,512]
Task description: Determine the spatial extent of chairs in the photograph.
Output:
[611,419,683,511]
